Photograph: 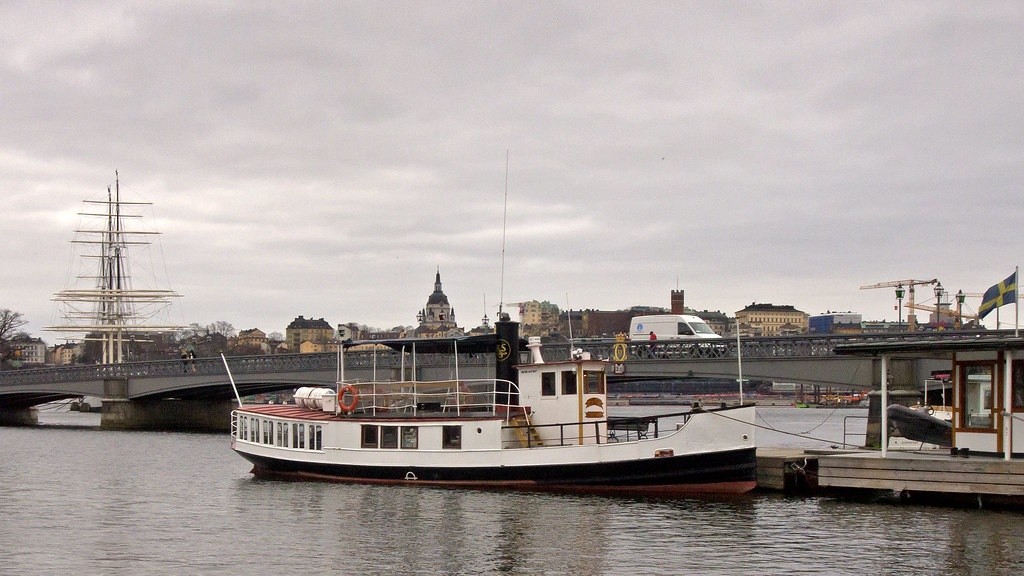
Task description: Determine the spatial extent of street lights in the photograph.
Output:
[955,289,965,339]
[934,282,944,332]
[895,283,905,333]
[482,314,490,335]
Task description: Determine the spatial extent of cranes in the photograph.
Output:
[905,289,1024,331]
[860,278,940,334]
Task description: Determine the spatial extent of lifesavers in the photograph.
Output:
[338,386,358,410]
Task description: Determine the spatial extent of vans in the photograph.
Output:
[628,313,728,357]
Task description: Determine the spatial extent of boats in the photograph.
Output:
[908,372,992,421]
[219,310,760,504]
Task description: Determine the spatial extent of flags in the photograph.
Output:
[977,271,1017,320]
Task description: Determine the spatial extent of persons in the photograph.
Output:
[399,330,407,339]
[648,331,658,358]
[181,350,197,375]
